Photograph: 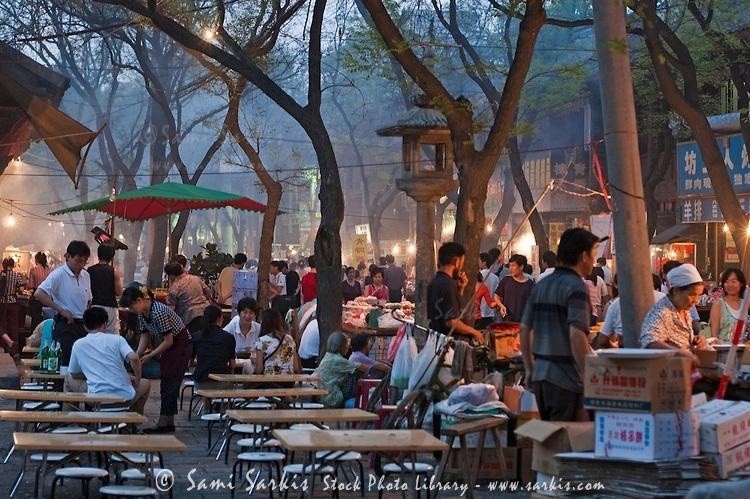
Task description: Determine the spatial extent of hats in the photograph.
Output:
[667,263,703,287]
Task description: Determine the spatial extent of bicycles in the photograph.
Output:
[375,306,527,476]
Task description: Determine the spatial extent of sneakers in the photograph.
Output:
[143,424,174,434]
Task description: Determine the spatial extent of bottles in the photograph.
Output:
[41,338,62,374]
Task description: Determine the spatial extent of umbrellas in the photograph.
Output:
[46,183,290,260]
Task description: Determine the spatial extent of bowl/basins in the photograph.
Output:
[236,353,251,359]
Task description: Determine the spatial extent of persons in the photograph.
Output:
[120,287,194,434]
[29,252,49,326]
[63,307,151,431]
[87,241,121,334]
[34,240,94,367]
[165,228,749,424]
[0,257,24,357]
